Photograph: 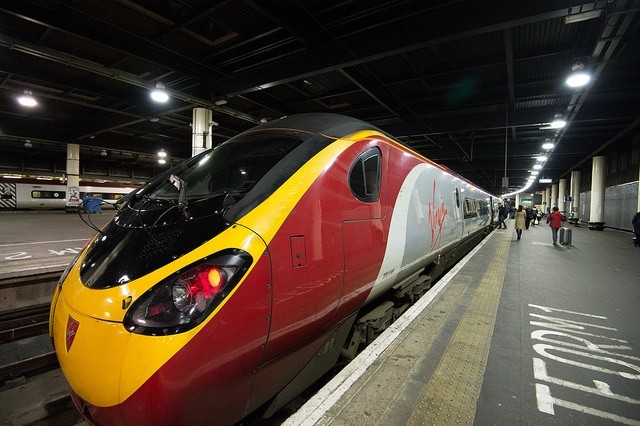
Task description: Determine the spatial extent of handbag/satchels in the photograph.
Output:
[529,214,534,219]
[536,213,542,218]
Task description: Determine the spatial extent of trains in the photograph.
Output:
[46,110,506,425]
[1,170,144,210]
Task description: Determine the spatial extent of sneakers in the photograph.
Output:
[497,226,501,229]
[553,241,556,245]
[502,227,507,229]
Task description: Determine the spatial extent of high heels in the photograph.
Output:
[517,234,520,240]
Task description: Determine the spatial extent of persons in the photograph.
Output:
[545,207,565,245]
[497,202,508,230]
[508,202,541,242]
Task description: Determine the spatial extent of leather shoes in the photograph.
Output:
[633,240,637,248]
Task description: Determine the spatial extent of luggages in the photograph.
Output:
[511,212,514,219]
[535,220,538,225]
[560,219,572,246]
